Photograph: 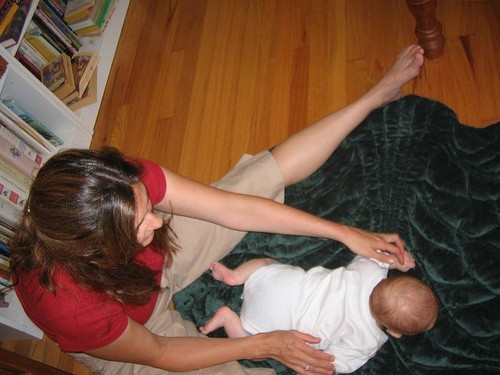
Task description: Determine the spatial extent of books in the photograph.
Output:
[0,96,63,308]
[0,0,119,111]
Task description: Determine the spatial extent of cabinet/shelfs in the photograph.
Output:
[0,0,131,340]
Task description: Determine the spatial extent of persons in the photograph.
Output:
[3,42,427,375]
[199,247,438,373]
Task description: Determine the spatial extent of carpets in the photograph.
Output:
[173,94,500,375]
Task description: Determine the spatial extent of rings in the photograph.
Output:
[304,364,311,372]
